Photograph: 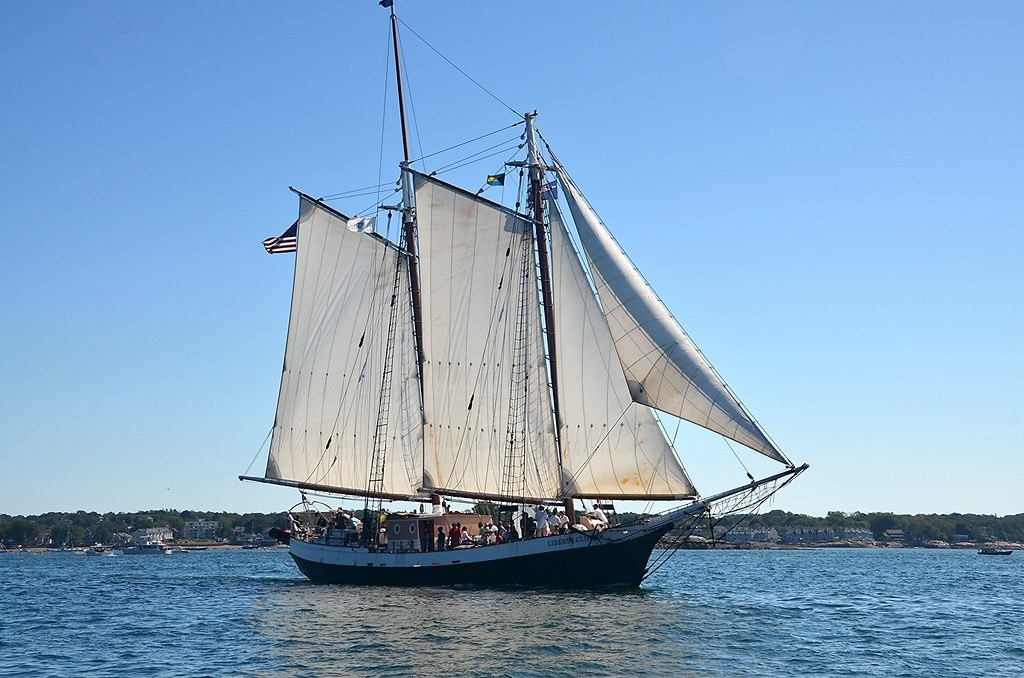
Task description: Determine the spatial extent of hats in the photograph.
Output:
[338,507,342,509]
[344,520,347,522]
[456,522,460,526]
[352,522,357,527]
[348,519,353,523]
[437,526,443,530]
[338,510,344,513]
[487,524,492,526]
[451,521,456,526]
[498,521,503,525]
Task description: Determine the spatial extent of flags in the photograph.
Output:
[261,220,298,254]
[487,174,505,187]
[347,216,376,233]
[526,178,557,201]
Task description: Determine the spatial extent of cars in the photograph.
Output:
[977,547,1013,555]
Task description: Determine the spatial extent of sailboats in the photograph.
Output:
[236,0,809,589]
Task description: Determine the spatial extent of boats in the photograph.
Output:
[242,545,260,549]
[121,536,190,555]
[85,545,118,558]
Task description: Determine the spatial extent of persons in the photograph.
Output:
[586,504,610,528]
[295,513,309,540]
[436,504,569,551]
[315,507,387,539]
